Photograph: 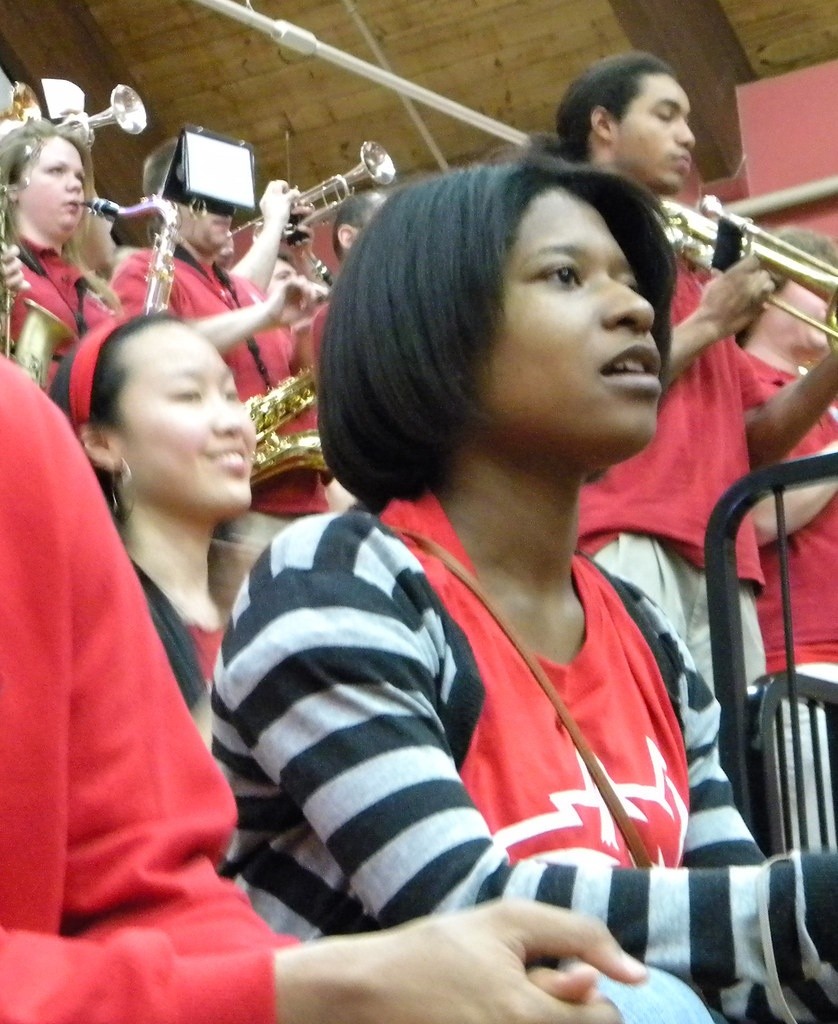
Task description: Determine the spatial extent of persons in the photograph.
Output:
[49,312,254,711]
[554,50,838,694]
[0,356,714,1024]
[109,137,317,398]
[736,226,838,849]
[314,191,387,364]
[0,109,118,391]
[212,164,838,1024]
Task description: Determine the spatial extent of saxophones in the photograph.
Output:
[0,188,70,391]
[245,353,336,492]
[82,191,186,317]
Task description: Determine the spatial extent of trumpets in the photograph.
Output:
[217,141,399,240]
[53,86,149,140]
[1,78,43,138]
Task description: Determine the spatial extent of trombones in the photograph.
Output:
[184,0,838,344]
